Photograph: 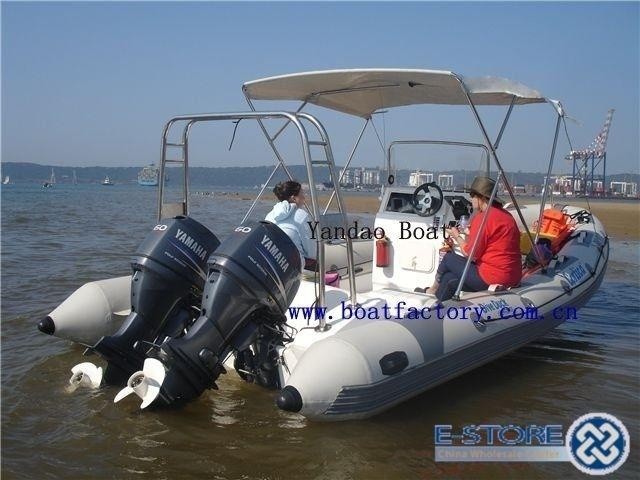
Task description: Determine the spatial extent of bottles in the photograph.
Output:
[376,239,390,267]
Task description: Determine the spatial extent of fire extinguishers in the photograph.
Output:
[374,228,389,267]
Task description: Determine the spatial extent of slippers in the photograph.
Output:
[414,287,430,293]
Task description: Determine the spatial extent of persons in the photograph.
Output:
[265,182,318,273]
[414,176,522,301]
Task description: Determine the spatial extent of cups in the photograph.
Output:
[460,215,469,231]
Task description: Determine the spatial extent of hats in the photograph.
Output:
[463,177,502,205]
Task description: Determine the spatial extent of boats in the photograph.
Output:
[35,67,612,424]
[135,163,169,186]
[101,175,114,186]
